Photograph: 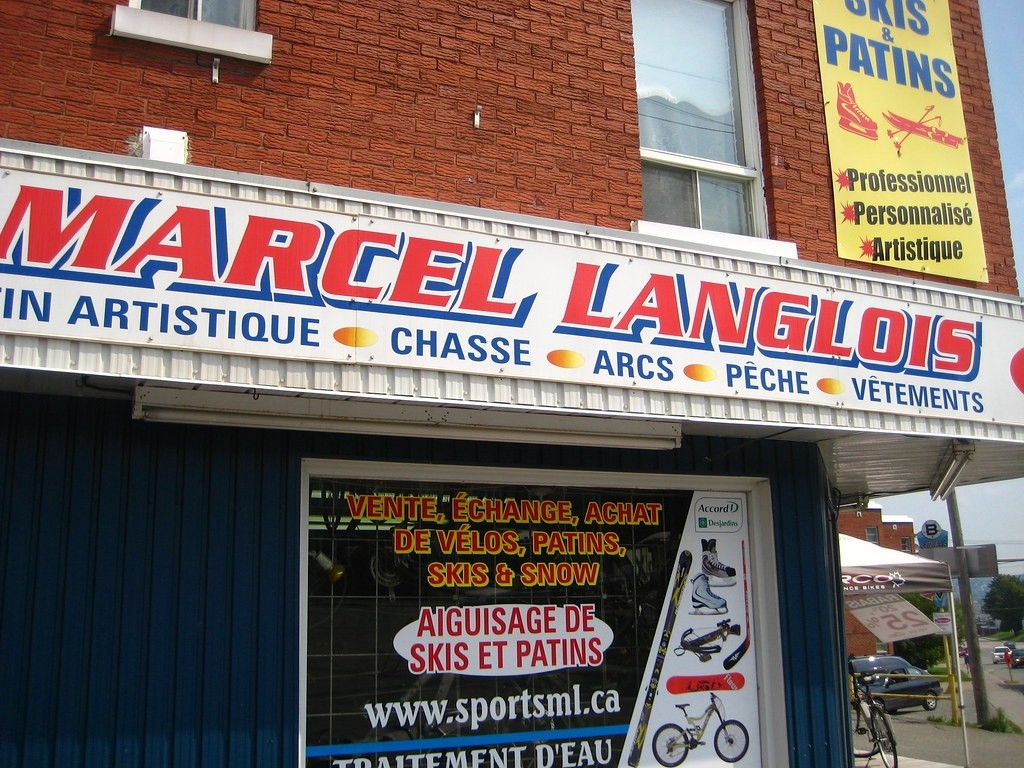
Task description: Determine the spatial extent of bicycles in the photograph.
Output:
[652,693,749,768]
[848,654,898,768]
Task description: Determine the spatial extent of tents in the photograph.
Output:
[837,532,971,768]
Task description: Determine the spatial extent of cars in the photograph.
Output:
[859,666,943,712]
[848,656,929,678]
[1010,649,1024,669]
[992,646,1012,664]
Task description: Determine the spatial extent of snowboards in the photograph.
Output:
[666,672,745,695]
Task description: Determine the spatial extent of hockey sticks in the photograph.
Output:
[723,541,751,671]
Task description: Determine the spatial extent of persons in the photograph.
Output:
[964,648,972,675]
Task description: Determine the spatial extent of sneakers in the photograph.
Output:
[689,573,729,614]
[700,539,737,587]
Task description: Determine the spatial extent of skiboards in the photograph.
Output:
[626,549,694,768]
